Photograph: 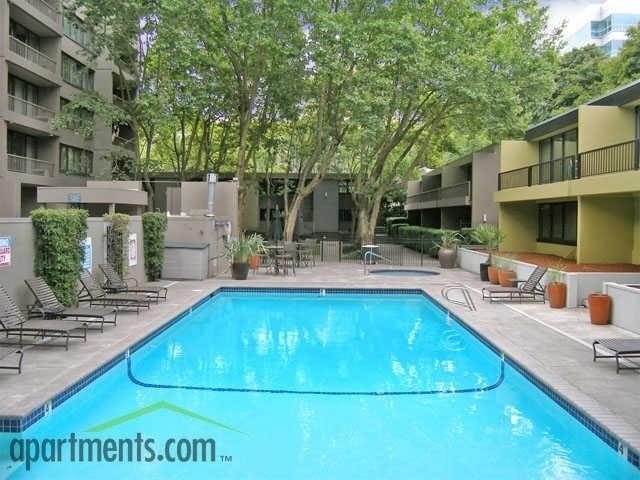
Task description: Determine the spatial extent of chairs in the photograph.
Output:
[593,338,640,374]
[251,238,319,277]
[481,264,548,304]
[0,264,167,374]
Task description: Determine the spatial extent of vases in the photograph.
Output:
[588,293,611,325]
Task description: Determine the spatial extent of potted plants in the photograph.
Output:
[431,225,468,268]
[469,223,508,281]
[220,229,271,280]
[546,257,566,308]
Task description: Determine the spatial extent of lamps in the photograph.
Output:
[206,172,218,216]
